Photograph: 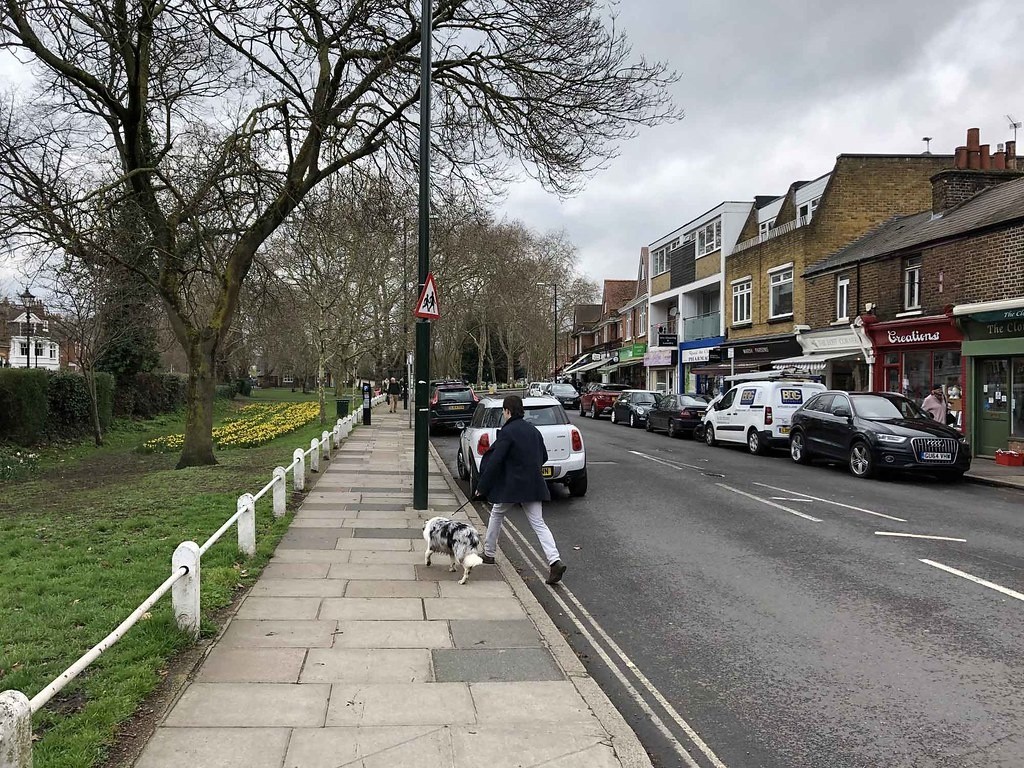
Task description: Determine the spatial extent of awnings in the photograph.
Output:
[598,361,641,373]
[559,354,612,374]
[690,361,774,375]
[771,352,863,369]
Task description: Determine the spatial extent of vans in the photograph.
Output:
[703,381,827,456]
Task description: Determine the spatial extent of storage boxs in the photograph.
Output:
[995,453,1024,466]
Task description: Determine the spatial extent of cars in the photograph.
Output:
[611,389,664,428]
[578,383,633,419]
[429,380,480,435]
[455,392,588,498]
[644,393,712,437]
[526,381,581,409]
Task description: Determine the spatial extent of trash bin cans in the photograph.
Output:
[336,399,350,418]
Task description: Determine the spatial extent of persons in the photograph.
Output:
[384,377,401,413]
[922,384,948,424]
[475,396,567,584]
[563,377,582,389]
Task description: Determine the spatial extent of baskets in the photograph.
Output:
[996,451,1024,466]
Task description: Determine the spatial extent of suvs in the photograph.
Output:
[788,390,971,482]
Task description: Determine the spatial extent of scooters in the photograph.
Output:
[693,394,722,442]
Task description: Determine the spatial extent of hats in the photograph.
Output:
[933,383,942,389]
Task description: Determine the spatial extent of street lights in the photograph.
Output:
[403,215,439,410]
[34,331,40,369]
[19,287,36,368]
[537,282,557,383]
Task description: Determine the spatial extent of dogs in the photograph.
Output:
[422,517,482,585]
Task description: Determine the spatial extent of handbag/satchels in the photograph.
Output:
[943,394,957,425]
[385,396,390,405]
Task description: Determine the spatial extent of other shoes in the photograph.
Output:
[389,411,392,413]
[545,561,566,584]
[479,552,495,564]
[394,410,396,412]
